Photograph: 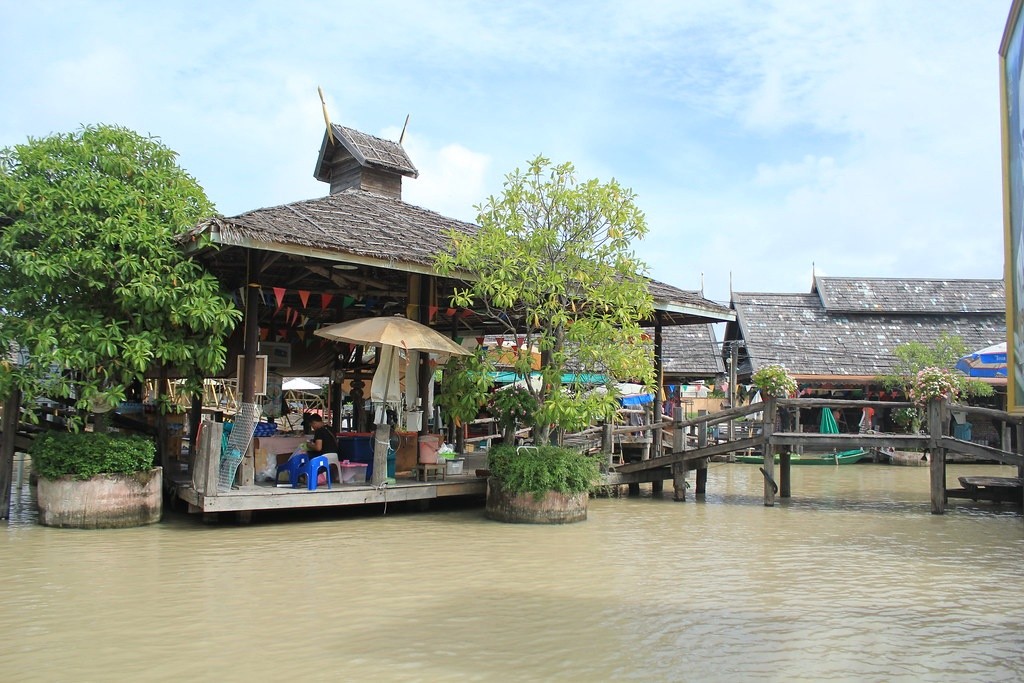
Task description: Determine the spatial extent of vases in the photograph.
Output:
[759,387,777,403]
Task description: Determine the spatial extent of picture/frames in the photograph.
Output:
[236,355,267,395]
[258,342,290,367]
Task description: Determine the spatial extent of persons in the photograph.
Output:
[307,413,339,474]
[859,407,874,435]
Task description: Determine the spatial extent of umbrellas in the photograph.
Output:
[744,390,764,456]
[282,378,322,399]
[169,378,222,385]
[495,374,576,398]
[579,382,655,463]
[955,342,1007,379]
[484,340,541,371]
[313,313,475,423]
[820,407,839,465]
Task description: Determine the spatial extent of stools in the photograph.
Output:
[415,464,447,481]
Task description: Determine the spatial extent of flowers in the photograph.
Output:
[750,363,798,399]
[909,366,960,408]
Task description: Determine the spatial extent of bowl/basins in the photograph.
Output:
[440,452,456,458]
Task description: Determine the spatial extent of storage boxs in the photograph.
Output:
[438,458,465,475]
[339,461,369,483]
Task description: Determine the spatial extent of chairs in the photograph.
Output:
[273,454,309,488]
[293,456,332,490]
[321,453,343,484]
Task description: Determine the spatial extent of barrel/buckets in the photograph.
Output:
[418,435,439,464]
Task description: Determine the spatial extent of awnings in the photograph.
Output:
[488,372,607,384]
